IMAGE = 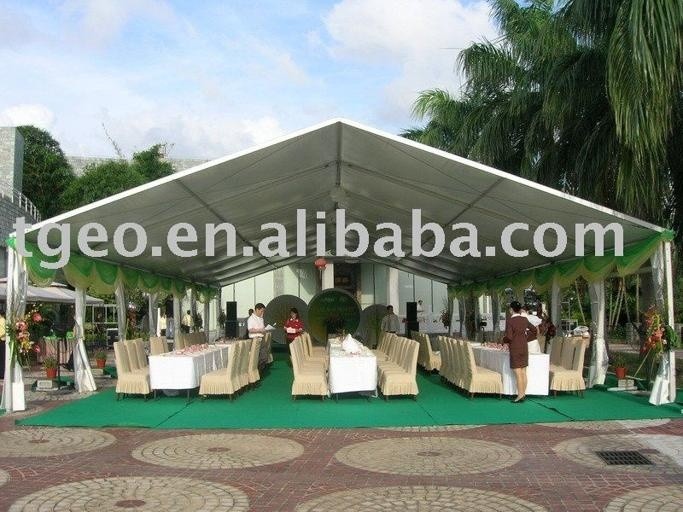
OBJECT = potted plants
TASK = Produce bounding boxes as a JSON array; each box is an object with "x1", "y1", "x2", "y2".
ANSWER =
[
  {"x1": 94, "y1": 351, "x2": 106, "y2": 369},
  {"x1": 42, "y1": 357, "x2": 60, "y2": 378},
  {"x1": 612, "y1": 351, "x2": 628, "y2": 378}
]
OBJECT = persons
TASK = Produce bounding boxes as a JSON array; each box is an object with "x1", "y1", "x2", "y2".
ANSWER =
[
  {"x1": 417, "y1": 300, "x2": 423, "y2": 317},
  {"x1": 501, "y1": 301, "x2": 536, "y2": 403},
  {"x1": 380, "y1": 305, "x2": 400, "y2": 333},
  {"x1": 248, "y1": 309, "x2": 253, "y2": 315},
  {"x1": 284, "y1": 308, "x2": 304, "y2": 366},
  {"x1": 180, "y1": 310, "x2": 194, "y2": 333},
  {"x1": 521, "y1": 306, "x2": 543, "y2": 352},
  {"x1": 247, "y1": 303, "x2": 268, "y2": 373},
  {"x1": 160, "y1": 311, "x2": 167, "y2": 336},
  {"x1": 1, "y1": 310, "x2": 6, "y2": 379}
]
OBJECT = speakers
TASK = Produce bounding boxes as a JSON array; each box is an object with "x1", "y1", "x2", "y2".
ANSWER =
[
  {"x1": 405, "y1": 322, "x2": 419, "y2": 338},
  {"x1": 224, "y1": 321, "x2": 239, "y2": 338},
  {"x1": 406, "y1": 301, "x2": 417, "y2": 321},
  {"x1": 226, "y1": 302, "x2": 237, "y2": 321}
]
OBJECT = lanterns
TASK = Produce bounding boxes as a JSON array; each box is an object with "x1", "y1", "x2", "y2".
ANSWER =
[{"x1": 314, "y1": 257, "x2": 326, "y2": 270}]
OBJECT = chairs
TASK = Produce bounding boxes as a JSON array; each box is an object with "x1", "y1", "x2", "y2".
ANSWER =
[{"x1": 111, "y1": 319, "x2": 594, "y2": 403}]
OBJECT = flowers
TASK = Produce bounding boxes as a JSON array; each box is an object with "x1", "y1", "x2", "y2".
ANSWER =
[
  {"x1": 640, "y1": 305, "x2": 681, "y2": 357},
  {"x1": 7, "y1": 295, "x2": 54, "y2": 365}
]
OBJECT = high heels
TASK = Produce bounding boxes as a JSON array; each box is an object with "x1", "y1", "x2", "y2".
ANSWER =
[{"x1": 509, "y1": 394, "x2": 527, "y2": 403}]
